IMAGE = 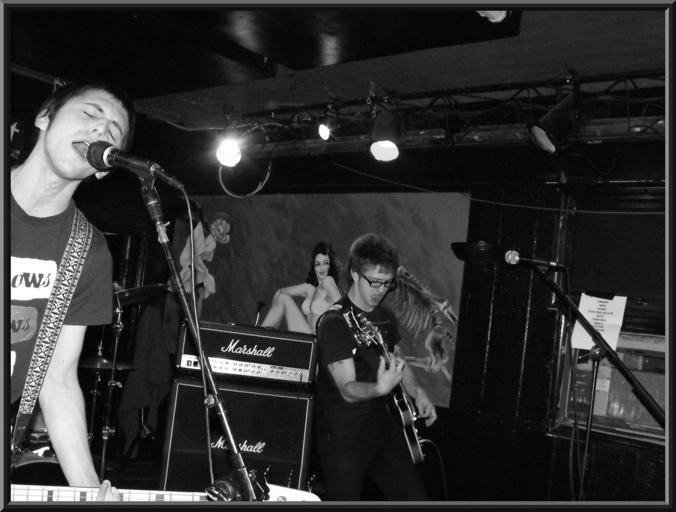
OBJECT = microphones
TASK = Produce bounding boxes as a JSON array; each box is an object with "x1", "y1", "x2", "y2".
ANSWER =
[
  {"x1": 86, "y1": 140, "x2": 161, "y2": 178},
  {"x1": 504, "y1": 250, "x2": 562, "y2": 270}
]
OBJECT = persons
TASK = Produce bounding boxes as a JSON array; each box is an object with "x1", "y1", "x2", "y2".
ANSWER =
[
  {"x1": 178, "y1": 211, "x2": 232, "y2": 322},
  {"x1": 260, "y1": 241, "x2": 343, "y2": 337},
  {"x1": 9, "y1": 76, "x2": 148, "y2": 503},
  {"x1": 310, "y1": 234, "x2": 439, "y2": 500}
]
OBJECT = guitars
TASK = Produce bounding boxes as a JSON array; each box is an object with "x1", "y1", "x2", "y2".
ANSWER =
[
  {"x1": 343, "y1": 306, "x2": 432, "y2": 464},
  {"x1": 10, "y1": 453, "x2": 323, "y2": 503}
]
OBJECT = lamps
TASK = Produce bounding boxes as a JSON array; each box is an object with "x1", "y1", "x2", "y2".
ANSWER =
[{"x1": 175, "y1": 66, "x2": 665, "y2": 202}]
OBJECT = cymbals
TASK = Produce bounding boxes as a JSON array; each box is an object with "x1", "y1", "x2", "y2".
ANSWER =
[
  {"x1": 112, "y1": 283, "x2": 170, "y2": 307},
  {"x1": 80, "y1": 357, "x2": 134, "y2": 371}
]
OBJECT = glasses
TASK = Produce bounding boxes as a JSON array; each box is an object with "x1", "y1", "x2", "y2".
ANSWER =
[{"x1": 358, "y1": 270, "x2": 397, "y2": 289}]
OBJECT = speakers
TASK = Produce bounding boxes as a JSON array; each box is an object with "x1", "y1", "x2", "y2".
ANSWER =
[{"x1": 157, "y1": 377, "x2": 317, "y2": 493}]
